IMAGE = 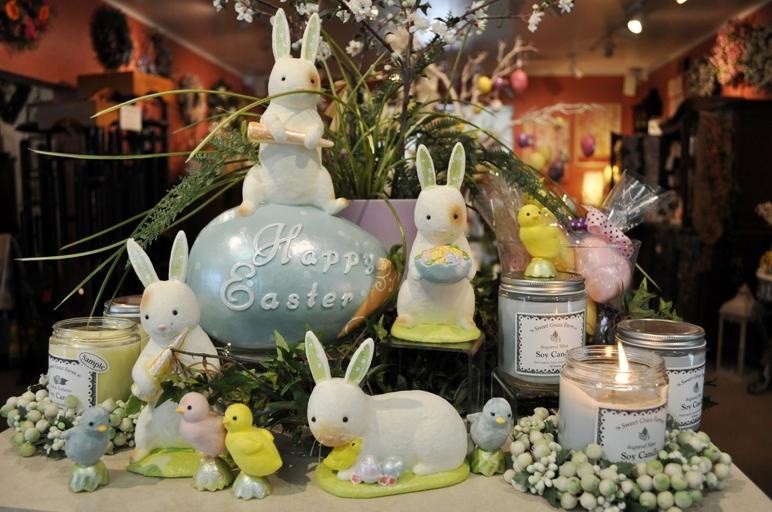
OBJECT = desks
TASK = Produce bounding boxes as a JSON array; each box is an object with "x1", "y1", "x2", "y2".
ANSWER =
[{"x1": 0, "y1": 424, "x2": 771, "y2": 512}]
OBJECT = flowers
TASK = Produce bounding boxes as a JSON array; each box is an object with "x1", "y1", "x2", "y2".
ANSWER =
[
  {"x1": 175, "y1": 73, "x2": 207, "y2": 127},
  {"x1": 0, "y1": 0, "x2": 53, "y2": 52},
  {"x1": 709, "y1": 17, "x2": 770, "y2": 93},
  {"x1": 38, "y1": 1, "x2": 680, "y2": 324}
]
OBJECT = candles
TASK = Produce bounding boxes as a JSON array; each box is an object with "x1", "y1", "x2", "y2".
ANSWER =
[
  {"x1": 49, "y1": 328, "x2": 140, "y2": 415},
  {"x1": 556, "y1": 365, "x2": 666, "y2": 463}
]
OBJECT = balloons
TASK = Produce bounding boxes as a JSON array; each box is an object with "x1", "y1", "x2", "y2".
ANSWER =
[
  {"x1": 477, "y1": 75, "x2": 493, "y2": 93},
  {"x1": 580, "y1": 134, "x2": 596, "y2": 158},
  {"x1": 541, "y1": 144, "x2": 552, "y2": 163},
  {"x1": 529, "y1": 152, "x2": 546, "y2": 171},
  {"x1": 519, "y1": 133, "x2": 534, "y2": 149},
  {"x1": 511, "y1": 70, "x2": 528, "y2": 93},
  {"x1": 548, "y1": 160, "x2": 566, "y2": 181}
]
